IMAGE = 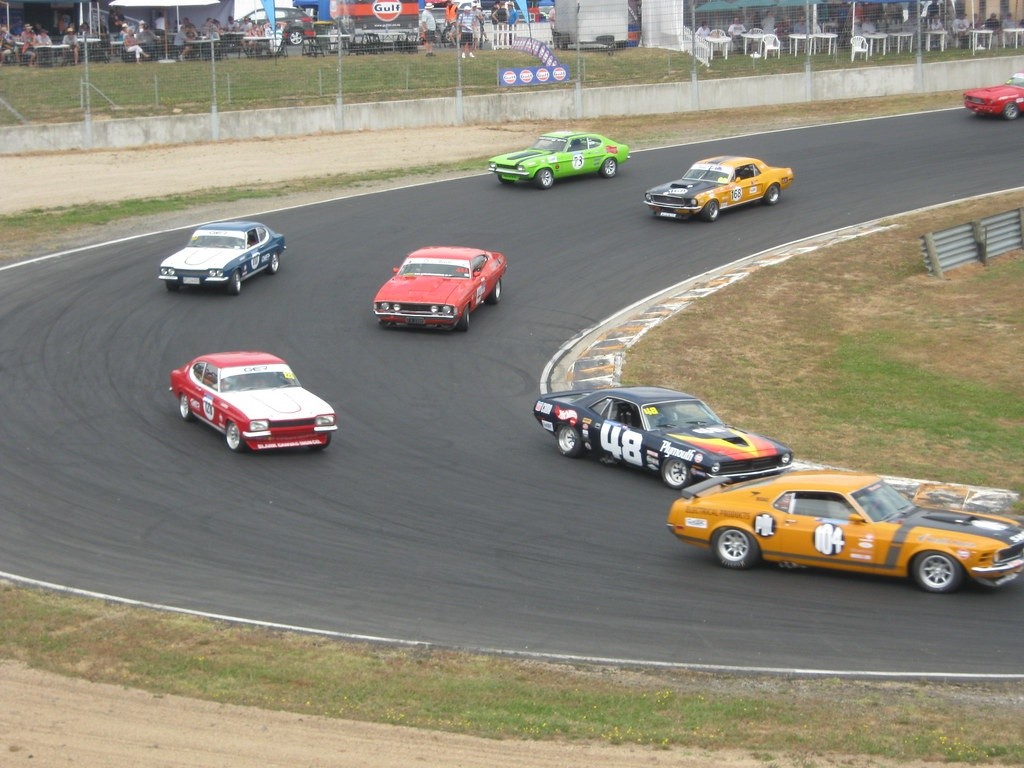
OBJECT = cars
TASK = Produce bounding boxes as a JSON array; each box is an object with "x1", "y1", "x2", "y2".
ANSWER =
[
  {"x1": 159, "y1": 221, "x2": 285, "y2": 295},
  {"x1": 963, "y1": 69, "x2": 1024, "y2": 121},
  {"x1": 418, "y1": 9, "x2": 464, "y2": 43},
  {"x1": 371, "y1": 247, "x2": 507, "y2": 331},
  {"x1": 532, "y1": 385, "x2": 794, "y2": 490},
  {"x1": 487, "y1": 130, "x2": 630, "y2": 189},
  {"x1": 169, "y1": 350, "x2": 337, "y2": 453},
  {"x1": 644, "y1": 155, "x2": 793, "y2": 223},
  {"x1": 666, "y1": 466, "x2": 1024, "y2": 593}
]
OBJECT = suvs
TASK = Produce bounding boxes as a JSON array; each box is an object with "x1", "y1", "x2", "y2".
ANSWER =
[{"x1": 235, "y1": 7, "x2": 317, "y2": 45}]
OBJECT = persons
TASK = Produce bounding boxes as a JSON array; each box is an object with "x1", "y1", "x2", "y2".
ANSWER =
[
  {"x1": 174, "y1": 13, "x2": 253, "y2": 60},
  {"x1": 697, "y1": 21, "x2": 710, "y2": 37},
  {"x1": 155, "y1": 12, "x2": 165, "y2": 34},
  {"x1": 79, "y1": 6, "x2": 154, "y2": 64},
  {"x1": 762, "y1": 11, "x2": 817, "y2": 52},
  {"x1": 728, "y1": 18, "x2": 752, "y2": 53},
  {"x1": 837, "y1": 2, "x2": 902, "y2": 47},
  {"x1": 0, "y1": 20, "x2": 78, "y2": 65},
  {"x1": 445, "y1": 1, "x2": 519, "y2": 58},
  {"x1": 422, "y1": 3, "x2": 436, "y2": 56},
  {"x1": 926, "y1": 0, "x2": 1024, "y2": 50}
]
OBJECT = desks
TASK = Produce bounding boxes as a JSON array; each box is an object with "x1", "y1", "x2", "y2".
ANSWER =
[
  {"x1": 923, "y1": 30, "x2": 949, "y2": 53},
  {"x1": 968, "y1": 29, "x2": 994, "y2": 51},
  {"x1": 220, "y1": 32, "x2": 246, "y2": 59},
  {"x1": 187, "y1": 39, "x2": 221, "y2": 58},
  {"x1": 891, "y1": 32, "x2": 913, "y2": 54},
  {"x1": 1002, "y1": 28, "x2": 1024, "y2": 49},
  {"x1": 375, "y1": 32, "x2": 405, "y2": 54},
  {"x1": 706, "y1": 36, "x2": 732, "y2": 61},
  {"x1": 740, "y1": 33, "x2": 765, "y2": 55},
  {"x1": 243, "y1": 36, "x2": 275, "y2": 59},
  {"x1": 862, "y1": 32, "x2": 888, "y2": 56},
  {"x1": 316, "y1": 34, "x2": 351, "y2": 54},
  {"x1": 813, "y1": 33, "x2": 839, "y2": 56},
  {"x1": 33, "y1": 45, "x2": 71, "y2": 66},
  {"x1": 77, "y1": 37, "x2": 102, "y2": 63},
  {"x1": 788, "y1": 33, "x2": 813, "y2": 57}
]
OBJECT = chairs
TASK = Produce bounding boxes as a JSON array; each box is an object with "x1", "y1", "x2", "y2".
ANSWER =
[
  {"x1": 808, "y1": 494, "x2": 832, "y2": 518},
  {"x1": 618, "y1": 403, "x2": 641, "y2": 428},
  {"x1": 0, "y1": 31, "x2": 421, "y2": 69},
  {"x1": 248, "y1": 235, "x2": 256, "y2": 246},
  {"x1": 708, "y1": 19, "x2": 1014, "y2": 66},
  {"x1": 569, "y1": 139, "x2": 585, "y2": 151}
]
